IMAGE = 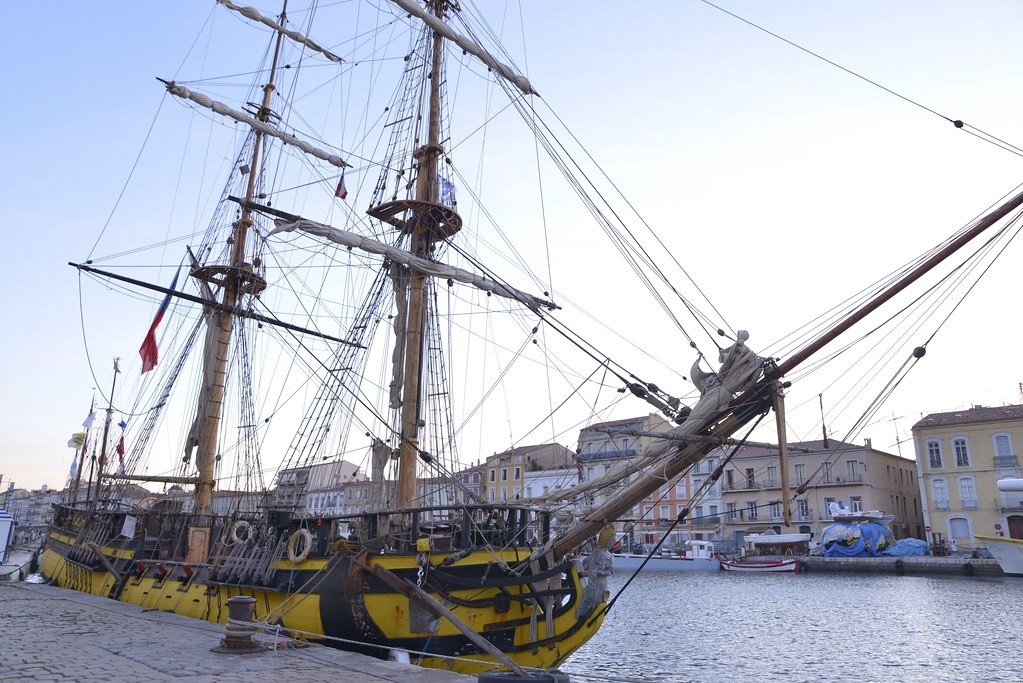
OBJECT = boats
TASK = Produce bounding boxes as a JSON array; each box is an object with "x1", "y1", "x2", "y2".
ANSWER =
[
  {"x1": 973, "y1": 535, "x2": 1023, "y2": 575},
  {"x1": 577, "y1": 540, "x2": 721, "y2": 572},
  {"x1": 718, "y1": 556, "x2": 800, "y2": 572}
]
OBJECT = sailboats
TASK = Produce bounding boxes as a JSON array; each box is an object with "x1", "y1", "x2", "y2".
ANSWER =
[{"x1": 37, "y1": 0, "x2": 1023, "y2": 675}]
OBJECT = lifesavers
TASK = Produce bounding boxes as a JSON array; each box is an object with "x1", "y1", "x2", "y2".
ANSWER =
[
  {"x1": 231, "y1": 521, "x2": 253, "y2": 545},
  {"x1": 287, "y1": 528, "x2": 312, "y2": 562}
]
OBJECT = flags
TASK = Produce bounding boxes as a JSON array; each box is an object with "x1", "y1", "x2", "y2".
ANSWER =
[{"x1": 138, "y1": 260, "x2": 184, "y2": 375}]
